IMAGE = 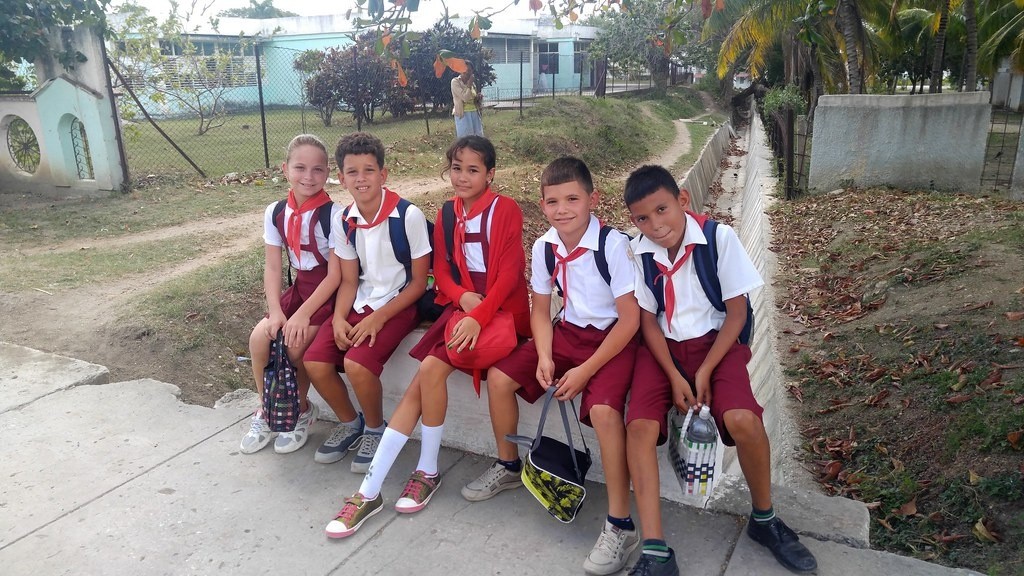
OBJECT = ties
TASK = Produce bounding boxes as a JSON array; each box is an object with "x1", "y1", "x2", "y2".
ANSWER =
[
  {"x1": 654, "y1": 211, "x2": 710, "y2": 334},
  {"x1": 286, "y1": 188, "x2": 332, "y2": 272},
  {"x1": 549, "y1": 218, "x2": 605, "y2": 329},
  {"x1": 339, "y1": 187, "x2": 403, "y2": 247},
  {"x1": 453, "y1": 185, "x2": 497, "y2": 293}
]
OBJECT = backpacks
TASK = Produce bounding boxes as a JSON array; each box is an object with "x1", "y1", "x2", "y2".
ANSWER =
[
  {"x1": 638, "y1": 219, "x2": 756, "y2": 348},
  {"x1": 341, "y1": 198, "x2": 446, "y2": 322}
]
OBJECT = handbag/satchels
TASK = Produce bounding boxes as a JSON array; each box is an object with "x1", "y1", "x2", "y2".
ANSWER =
[
  {"x1": 262, "y1": 327, "x2": 300, "y2": 432},
  {"x1": 667, "y1": 401, "x2": 719, "y2": 494},
  {"x1": 503, "y1": 375, "x2": 592, "y2": 523},
  {"x1": 445, "y1": 295, "x2": 517, "y2": 369}
]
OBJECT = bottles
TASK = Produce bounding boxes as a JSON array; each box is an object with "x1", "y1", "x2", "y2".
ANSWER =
[{"x1": 688, "y1": 405, "x2": 716, "y2": 444}]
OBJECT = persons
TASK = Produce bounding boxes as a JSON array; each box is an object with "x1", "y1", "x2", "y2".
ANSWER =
[
  {"x1": 460, "y1": 156, "x2": 641, "y2": 576},
  {"x1": 236, "y1": 134, "x2": 342, "y2": 455},
  {"x1": 325, "y1": 135, "x2": 531, "y2": 539},
  {"x1": 623, "y1": 164, "x2": 818, "y2": 576},
  {"x1": 451, "y1": 59, "x2": 485, "y2": 138},
  {"x1": 300, "y1": 131, "x2": 431, "y2": 475}
]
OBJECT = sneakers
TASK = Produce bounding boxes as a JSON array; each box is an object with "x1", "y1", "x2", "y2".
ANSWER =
[
  {"x1": 324, "y1": 491, "x2": 384, "y2": 539},
  {"x1": 747, "y1": 516, "x2": 819, "y2": 573},
  {"x1": 583, "y1": 517, "x2": 640, "y2": 576},
  {"x1": 459, "y1": 460, "x2": 524, "y2": 502},
  {"x1": 314, "y1": 411, "x2": 365, "y2": 463},
  {"x1": 350, "y1": 420, "x2": 389, "y2": 474},
  {"x1": 274, "y1": 396, "x2": 319, "y2": 453},
  {"x1": 394, "y1": 469, "x2": 442, "y2": 513},
  {"x1": 628, "y1": 547, "x2": 680, "y2": 576},
  {"x1": 240, "y1": 408, "x2": 277, "y2": 454}
]
